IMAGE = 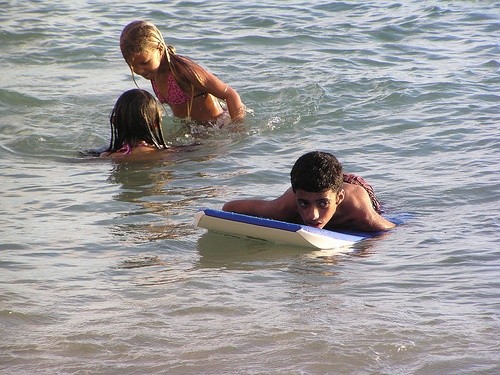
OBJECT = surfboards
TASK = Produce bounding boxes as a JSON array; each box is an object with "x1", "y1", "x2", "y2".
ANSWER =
[{"x1": 194, "y1": 209, "x2": 373, "y2": 249}]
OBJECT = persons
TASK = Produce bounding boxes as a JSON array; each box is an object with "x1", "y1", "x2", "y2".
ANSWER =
[
  {"x1": 77, "y1": 88, "x2": 176, "y2": 161},
  {"x1": 222, "y1": 151, "x2": 416, "y2": 235},
  {"x1": 120, "y1": 19, "x2": 245, "y2": 130}
]
